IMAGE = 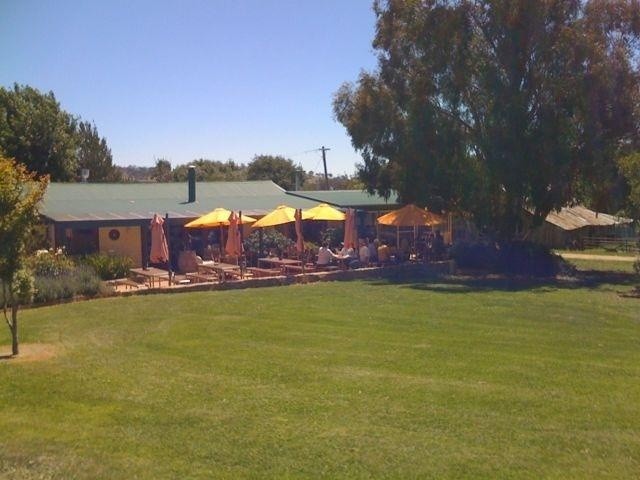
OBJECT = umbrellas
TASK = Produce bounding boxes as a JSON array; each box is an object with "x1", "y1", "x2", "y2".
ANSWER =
[
  {"x1": 305, "y1": 202, "x2": 345, "y2": 244},
  {"x1": 376, "y1": 203, "x2": 448, "y2": 246},
  {"x1": 344, "y1": 207, "x2": 360, "y2": 250},
  {"x1": 147, "y1": 214, "x2": 171, "y2": 264},
  {"x1": 184, "y1": 208, "x2": 257, "y2": 257},
  {"x1": 226, "y1": 211, "x2": 242, "y2": 259},
  {"x1": 294, "y1": 209, "x2": 305, "y2": 256},
  {"x1": 250, "y1": 205, "x2": 313, "y2": 258}
]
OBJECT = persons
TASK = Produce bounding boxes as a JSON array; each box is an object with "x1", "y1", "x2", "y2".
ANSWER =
[{"x1": 315, "y1": 230, "x2": 446, "y2": 271}]
